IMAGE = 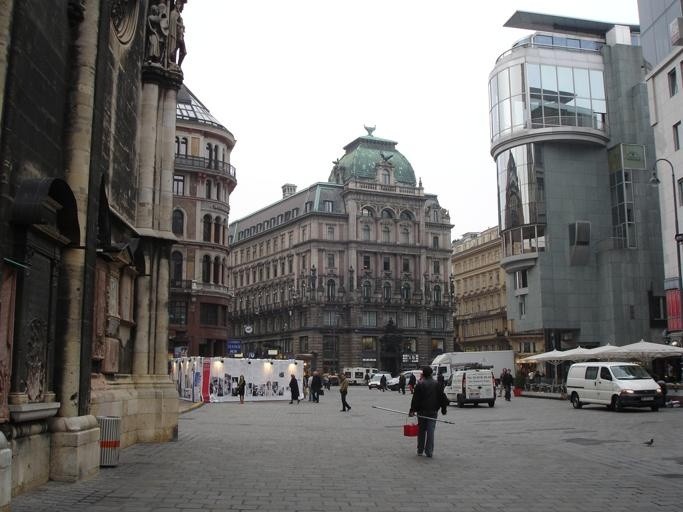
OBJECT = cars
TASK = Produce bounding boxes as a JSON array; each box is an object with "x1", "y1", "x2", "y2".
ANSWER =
[{"x1": 340, "y1": 366, "x2": 422, "y2": 390}]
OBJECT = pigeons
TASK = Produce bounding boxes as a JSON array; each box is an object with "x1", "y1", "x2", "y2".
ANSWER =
[{"x1": 643, "y1": 438, "x2": 654, "y2": 447}]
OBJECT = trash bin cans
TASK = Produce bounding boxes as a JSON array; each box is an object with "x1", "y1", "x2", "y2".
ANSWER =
[{"x1": 96, "y1": 415, "x2": 121, "y2": 468}]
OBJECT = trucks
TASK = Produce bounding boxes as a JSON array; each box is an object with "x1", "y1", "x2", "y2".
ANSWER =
[{"x1": 431, "y1": 351, "x2": 516, "y2": 382}]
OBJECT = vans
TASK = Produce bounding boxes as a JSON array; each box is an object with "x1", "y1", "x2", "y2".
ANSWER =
[
  {"x1": 566, "y1": 362, "x2": 664, "y2": 412},
  {"x1": 444, "y1": 370, "x2": 496, "y2": 408}
]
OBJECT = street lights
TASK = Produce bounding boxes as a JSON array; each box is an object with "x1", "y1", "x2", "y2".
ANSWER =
[{"x1": 650, "y1": 158, "x2": 682, "y2": 310}]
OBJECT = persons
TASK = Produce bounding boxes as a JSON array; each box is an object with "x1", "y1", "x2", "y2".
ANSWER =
[
  {"x1": 212, "y1": 378, "x2": 219, "y2": 397},
  {"x1": 534, "y1": 370, "x2": 545, "y2": 384},
  {"x1": 233, "y1": 378, "x2": 238, "y2": 393},
  {"x1": 497, "y1": 368, "x2": 507, "y2": 397},
  {"x1": 503, "y1": 368, "x2": 514, "y2": 401},
  {"x1": 666, "y1": 362, "x2": 674, "y2": 382},
  {"x1": 408, "y1": 366, "x2": 448, "y2": 458},
  {"x1": 365, "y1": 371, "x2": 370, "y2": 385},
  {"x1": 264, "y1": 381, "x2": 277, "y2": 397},
  {"x1": 288, "y1": 370, "x2": 332, "y2": 404},
  {"x1": 398, "y1": 371, "x2": 445, "y2": 394},
  {"x1": 339, "y1": 372, "x2": 351, "y2": 412},
  {"x1": 237, "y1": 374, "x2": 246, "y2": 404},
  {"x1": 380, "y1": 374, "x2": 387, "y2": 392},
  {"x1": 528, "y1": 369, "x2": 535, "y2": 382}
]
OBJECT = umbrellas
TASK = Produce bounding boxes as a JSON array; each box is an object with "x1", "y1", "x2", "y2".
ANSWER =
[
  {"x1": 591, "y1": 341, "x2": 619, "y2": 362},
  {"x1": 607, "y1": 337, "x2": 683, "y2": 366},
  {"x1": 536, "y1": 344, "x2": 596, "y2": 365},
  {"x1": 516, "y1": 348, "x2": 568, "y2": 385}
]
